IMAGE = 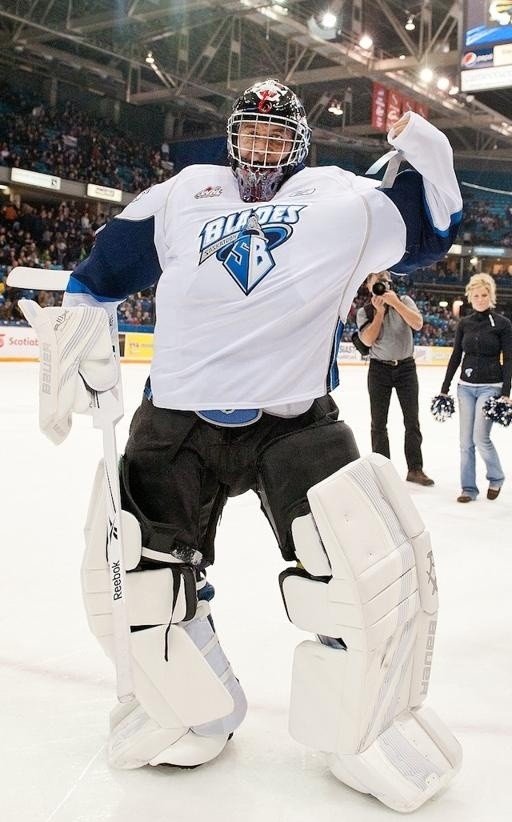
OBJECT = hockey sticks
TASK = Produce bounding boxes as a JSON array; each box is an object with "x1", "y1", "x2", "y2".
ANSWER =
[{"x1": 8, "y1": 268, "x2": 136, "y2": 717}]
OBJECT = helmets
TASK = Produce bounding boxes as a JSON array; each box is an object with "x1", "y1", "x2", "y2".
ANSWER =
[{"x1": 227, "y1": 80, "x2": 312, "y2": 166}]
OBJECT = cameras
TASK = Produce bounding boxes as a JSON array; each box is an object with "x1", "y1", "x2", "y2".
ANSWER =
[{"x1": 372, "y1": 281, "x2": 389, "y2": 296}]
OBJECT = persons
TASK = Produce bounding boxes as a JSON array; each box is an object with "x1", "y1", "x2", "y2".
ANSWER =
[
  {"x1": 438, "y1": 272, "x2": 512, "y2": 503},
  {"x1": 355, "y1": 268, "x2": 435, "y2": 486},
  {"x1": 18, "y1": 79, "x2": 465, "y2": 812},
  {"x1": 1, "y1": 90, "x2": 512, "y2": 346}
]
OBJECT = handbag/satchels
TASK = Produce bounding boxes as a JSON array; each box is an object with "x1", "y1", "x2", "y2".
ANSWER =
[{"x1": 352, "y1": 320, "x2": 369, "y2": 355}]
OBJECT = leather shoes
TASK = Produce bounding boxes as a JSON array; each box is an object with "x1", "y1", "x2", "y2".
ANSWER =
[{"x1": 406, "y1": 471, "x2": 434, "y2": 486}]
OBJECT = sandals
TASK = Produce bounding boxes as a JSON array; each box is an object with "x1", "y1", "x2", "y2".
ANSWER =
[
  {"x1": 488, "y1": 485, "x2": 500, "y2": 500},
  {"x1": 457, "y1": 496, "x2": 471, "y2": 503}
]
